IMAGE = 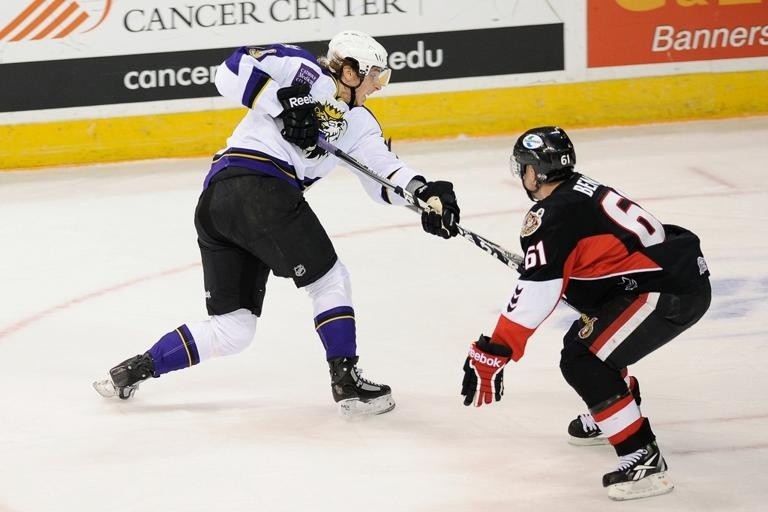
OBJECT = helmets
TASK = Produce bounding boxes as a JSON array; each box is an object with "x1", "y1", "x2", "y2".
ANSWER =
[
  {"x1": 510, "y1": 127, "x2": 576, "y2": 184},
  {"x1": 327, "y1": 31, "x2": 392, "y2": 87}
]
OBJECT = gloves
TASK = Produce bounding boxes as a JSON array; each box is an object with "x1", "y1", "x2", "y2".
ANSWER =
[
  {"x1": 415, "y1": 181, "x2": 459, "y2": 239},
  {"x1": 277, "y1": 85, "x2": 319, "y2": 150},
  {"x1": 461, "y1": 335, "x2": 512, "y2": 407}
]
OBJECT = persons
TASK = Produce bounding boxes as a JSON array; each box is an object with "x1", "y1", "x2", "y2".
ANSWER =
[
  {"x1": 110, "y1": 29, "x2": 456, "y2": 407},
  {"x1": 460, "y1": 126, "x2": 713, "y2": 489}
]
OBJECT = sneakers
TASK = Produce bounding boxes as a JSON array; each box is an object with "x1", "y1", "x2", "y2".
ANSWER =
[
  {"x1": 568, "y1": 406, "x2": 642, "y2": 438},
  {"x1": 603, "y1": 440, "x2": 667, "y2": 487}
]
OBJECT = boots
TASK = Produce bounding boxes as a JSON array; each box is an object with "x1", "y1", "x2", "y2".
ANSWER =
[
  {"x1": 110, "y1": 351, "x2": 152, "y2": 399},
  {"x1": 328, "y1": 356, "x2": 390, "y2": 402}
]
